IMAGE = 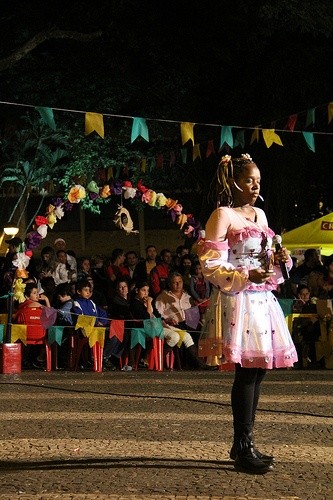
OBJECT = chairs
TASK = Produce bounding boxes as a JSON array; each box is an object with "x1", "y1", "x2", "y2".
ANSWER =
[{"x1": 11, "y1": 307, "x2": 175, "y2": 372}]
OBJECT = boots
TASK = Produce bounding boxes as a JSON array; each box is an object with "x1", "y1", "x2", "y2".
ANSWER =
[
  {"x1": 230, "y1": 421, "x2": 276, "y2": 463},
  {"x1": 234, "y1": 430, "x2": 268, "y2": 475}
]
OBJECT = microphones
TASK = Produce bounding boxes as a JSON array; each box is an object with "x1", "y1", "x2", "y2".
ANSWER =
[{"x1": 273, "y1": 235, "x2": 290, "y2": 280}]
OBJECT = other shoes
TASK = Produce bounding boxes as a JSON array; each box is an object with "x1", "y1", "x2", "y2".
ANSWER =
[
  {"x1": 121, "y1": 365, "x2": 126, "y2": 371},
  {"x1": 126, "y1": 366, "x2": 133, "y2": 371},
  {"x1": 204, "y1": 365, "x2": 218, "y2": 370},
  {"x1": 29, "y1": 363, "x2": 39, "y2": 369},
  {"x1": 112, "y1": 367, "x2": 118, "y2": 370}
]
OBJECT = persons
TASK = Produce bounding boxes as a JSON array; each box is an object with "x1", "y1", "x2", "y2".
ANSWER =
[
  {"x1": 198, "y1": 154, "x2": 299, "y2": 473},
  {"x1": 275, "y1": 248, "x2": 333, "y2": 370},
  {"x1": 0, "y1": 237, "x2": 210, "y2": 372}
]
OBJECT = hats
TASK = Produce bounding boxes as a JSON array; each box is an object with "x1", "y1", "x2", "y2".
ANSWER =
[{"x1": 54, "y1": 238, "x2": 65, "y2": 245}]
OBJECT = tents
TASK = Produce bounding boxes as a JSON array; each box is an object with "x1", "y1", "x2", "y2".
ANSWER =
[{"x1": 271, "y1": 211, "x2": 333, "y2": 256}]
